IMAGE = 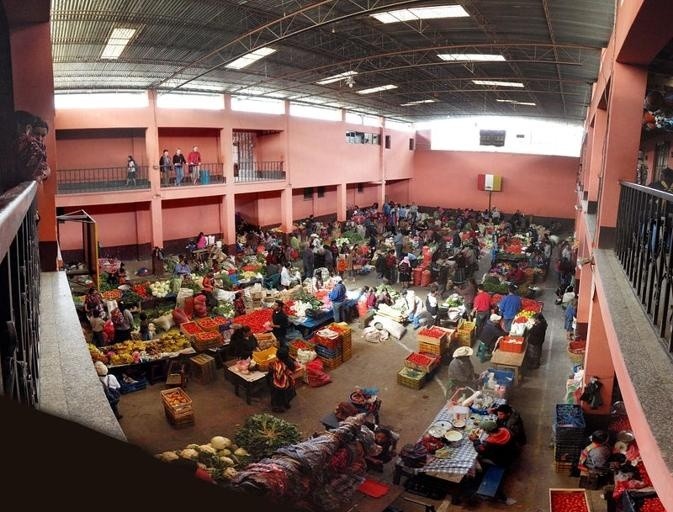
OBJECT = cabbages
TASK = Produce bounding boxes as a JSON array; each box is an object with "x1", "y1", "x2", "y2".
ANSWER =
[
  {"x1": 159, "y1": 436, "x2": 248, "y2": 480},
  {"x1": 149, "y1": 280, "x2": 172, "y2": 298}
]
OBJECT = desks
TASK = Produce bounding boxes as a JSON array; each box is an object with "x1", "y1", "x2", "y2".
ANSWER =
[
  {"x1": 225, "y1": 357, "x2": 271, "y2": 406},
  {"x1": 490, "y1": 342, "x2": 528, "y2": 385},
  {"x1": 319, "y1": 401, "x2": 366, "y2": 431},
  {"x1": 192, "y1": 249, "x2": 210, "y2": 261},
  {"x1": 393, "y1": 393, "x2": 508, "y2": 497},
  {"x1": 331, "y1": 477, "x2": 405, "y2": 512}
]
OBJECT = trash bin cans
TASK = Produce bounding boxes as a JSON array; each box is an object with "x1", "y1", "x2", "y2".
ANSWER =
[
  {"x1": 200, "y1": 170, "x2": 209, "y2": 184},
  {"x1": 152, "y1": 246, "x2": 164, "y2": 274}
]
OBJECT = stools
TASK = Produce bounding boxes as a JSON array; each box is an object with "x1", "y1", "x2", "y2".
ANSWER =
[{"x1": 475, "y1": 341, "x2": 490, "y2": 363}]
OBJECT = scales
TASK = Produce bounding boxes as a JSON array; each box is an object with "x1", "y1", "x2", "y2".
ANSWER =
[{"x1": 613, "y1": 429, "x2": 636, "y2": 455}]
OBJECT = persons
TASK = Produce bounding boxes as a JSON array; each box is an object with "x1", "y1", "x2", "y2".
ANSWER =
[
  {"x1": 602, "y1": 453, "x2": 642, "y2": 512},
  {"x1": 637, "y1": 150, "x2": 673, "y2": 258},
  {"x1": 172, "y1": 147, "x2": 186, "y2": 186},
  {"x1": 1, "y1": 111, "x2": 51, "y2": 195},
  {"x1": 32, "y1": 114, "x2": 49, "y2": 143},
  {"x1": 186, "y1": 145, "x2": 202, "y2": 184},
  {"x1": 125, "y1": 155, "x2": 138, "y2": 186},
  {"x1": 577, "y1": 430, "x2": 615, "y2": 488},
  {"x1": 160, "y1": 149, "x2": 173, "y2": 184},
  {"x1": 83, "y1": 199, "x2": 572, "y2": 469}
]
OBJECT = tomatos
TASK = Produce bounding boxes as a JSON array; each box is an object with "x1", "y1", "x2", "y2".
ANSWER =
[
  {"x1": 640, "y1": 497, "x2": 666, "y2": 512},
  {"x1": 135, "y1": 286, "x2": 145, "y2": 296},
  {"x1": 407, "y1": 354, "x2": 431, "y2": 365},
  {"x1": 552, "y1": 493, "x2": 588, "y2": 512},
  {"x1": 608, "y1": 415, "x2": 652, "y2": 490},
  {"x1": 489, "y1": 296, "x2": 541, "y2": 313},
  {"x1": 329, "y1": 325, "x2": 345, "y2": 333},
  {"x1": 183, "y1": 316, "x2": 226, "y2": 341},
  {"x1": 234, "y1": 308, "x2": 273, "y2": 334},
  {"x1": 420, "y1": 328, "x2": 442, "y2": 338},
  {"x1": 292, "y1": 340, "x2": 308, "y2": 348}
]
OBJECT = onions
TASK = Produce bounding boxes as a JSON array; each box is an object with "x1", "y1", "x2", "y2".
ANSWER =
[{"x1": 424, "y1": 433, "x2": 443, "y2": 451}]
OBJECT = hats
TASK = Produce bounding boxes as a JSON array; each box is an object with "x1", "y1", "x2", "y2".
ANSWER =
[
  {"x1": 95, "y1": 361, "x2": 108, "y2": 376},
  {"x1": 489, "y1": 314, "x2": 501, "y2": 321},
  {"x1": 480, "y1": 422, "x2": 497, "y2": 432}
]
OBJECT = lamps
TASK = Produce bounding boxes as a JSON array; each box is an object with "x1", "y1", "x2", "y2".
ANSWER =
[
  {"x1": 575, "y1": 253, "x2": 595, "y2": 269},
  {"x1": 575, "y1": 204, "x2": 582, "y2": 212}
]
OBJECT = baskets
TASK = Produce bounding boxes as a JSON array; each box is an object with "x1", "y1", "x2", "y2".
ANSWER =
[
  {"x1": 396, "y1": 322, "x2": 475, "y2": 390},
  {"x1": 119, "y1": 376, "x2": 145, "y2": 394},
  {"x1": 254, "y1": 322, "x2": 351, "y2": 371},
  {"x1": 552, "y1": 404, "x2": 585, "y2": 473},
  {"x1": 160, "y1": 388, "x2": 194, "y2": 430},
  {"x1": 181, "y1": 317, "x2": 231, "y2": 352}
]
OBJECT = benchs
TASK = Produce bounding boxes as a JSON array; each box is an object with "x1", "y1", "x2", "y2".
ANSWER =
[{"x1": 476, "y1": 467, "x2": 505, "y2": 498}]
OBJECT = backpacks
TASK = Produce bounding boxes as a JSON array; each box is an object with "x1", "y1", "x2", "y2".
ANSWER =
[{"x1": 99, "y1": 376, "x2": 119, "y2": 406}]
